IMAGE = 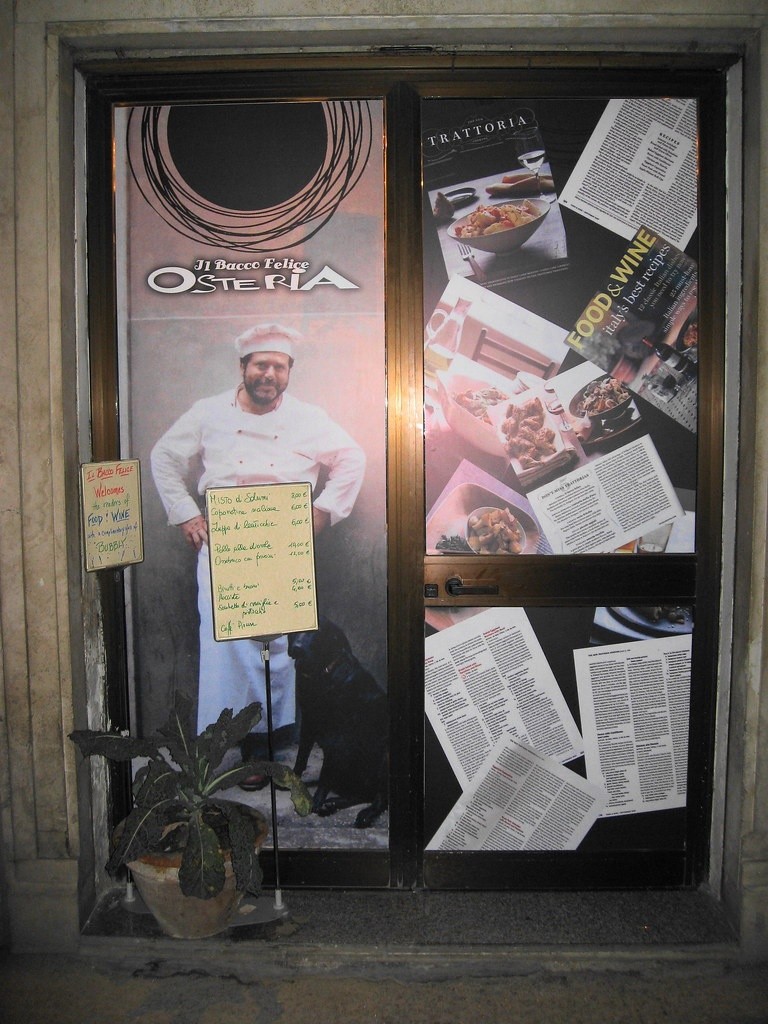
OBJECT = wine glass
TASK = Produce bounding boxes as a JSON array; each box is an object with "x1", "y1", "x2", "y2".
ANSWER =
[
  {"x1": 512, "y1": 127, "x2": 556, "y2": 203},
  {"x1": 544, "y1": 393, "x2": 573, "y2": 431},
  {"x1": 652, "y1": 367, "x2": 691, "y2": 398}
]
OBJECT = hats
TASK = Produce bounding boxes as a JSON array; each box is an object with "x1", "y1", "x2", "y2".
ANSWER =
[{"x1": 236, "y1": 322, "x2": 303, "y2": 357}]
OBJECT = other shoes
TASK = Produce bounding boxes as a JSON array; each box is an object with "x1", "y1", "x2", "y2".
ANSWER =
[{"x1": 239, "y1": 758, "x2": 267, "y2": 791}]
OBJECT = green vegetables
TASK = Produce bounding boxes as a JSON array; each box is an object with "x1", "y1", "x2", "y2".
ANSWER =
[{"x1": 435, "y1": 534, "x2": 473, "y2": 551}]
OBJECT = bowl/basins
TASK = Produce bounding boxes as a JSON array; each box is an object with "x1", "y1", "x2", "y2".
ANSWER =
[
  {"x1": 447, "y1": 198, "x2": 551, "y2": 256},
  {"x1": 436, "y1": 372, "x2": 510, "y2": 457},
  {"x1": 514, "y1": 372, "x2": 545, "y2": 392},
  {"x1": 676, "y1": 306, "x2": 697, "y2": 353},
  {"x1": 465, "y1": 507, "x2": 526, "y2": 555},
  {"x1": 569, "y1": 373, "x2": 633, "y2": 420}
]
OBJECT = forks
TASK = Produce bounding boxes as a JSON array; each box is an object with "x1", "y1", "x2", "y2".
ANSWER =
[{"x1": 458, "y1": 243, "x2": 488, "y2": 282}]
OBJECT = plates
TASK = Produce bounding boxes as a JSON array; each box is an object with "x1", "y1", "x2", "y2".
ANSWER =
[
  {"x1": 444, "y1": 187, "x2": 477, "y2": 208},
  {"x1": 610, "y1": 607, "x2": 693, "y2": 633}
]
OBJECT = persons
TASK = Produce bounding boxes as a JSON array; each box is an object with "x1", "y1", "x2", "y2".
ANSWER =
[{"x1": 149, "y1": 325, "x2": 367, "y2": 792}]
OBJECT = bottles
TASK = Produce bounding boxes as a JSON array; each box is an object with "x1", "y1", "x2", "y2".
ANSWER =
[
  {"x1": 642, "y1": 336, "x2": 697, "y2": 378},
  {"x1": 424, "y1": 297, "x2": 473, "y2": 379}
]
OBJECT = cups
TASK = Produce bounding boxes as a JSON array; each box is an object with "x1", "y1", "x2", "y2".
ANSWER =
[
  {"x1": 637, "y1": 522, "x2": 673, "y2": 553},
  {"x1": 614, "y1": 540, "x2": 635, "y2": 553}
]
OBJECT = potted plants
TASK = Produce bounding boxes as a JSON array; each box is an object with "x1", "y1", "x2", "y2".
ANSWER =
[{"x1": 106, "y1": 701, "x2": 316, "y2": 938}]
